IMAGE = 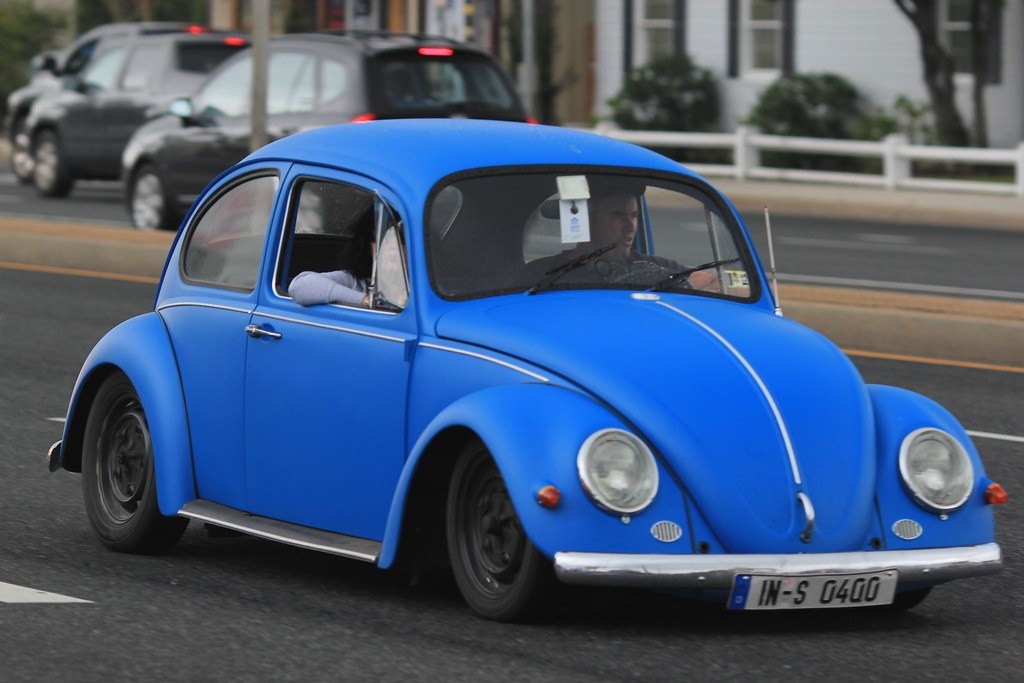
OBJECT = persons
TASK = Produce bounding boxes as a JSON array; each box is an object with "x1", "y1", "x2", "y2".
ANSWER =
[
  {"x1": 283, "y1": 202, "x2": 439, "y2": 309},
  {"x1": 518, "y1": 192, "x2": 716, "y2": 292}
]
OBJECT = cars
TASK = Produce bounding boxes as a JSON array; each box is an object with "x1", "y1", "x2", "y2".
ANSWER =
[
  {"x1": 3, "y1": 20, "x2": 210, "y2": 189},
  {"x1": 121, "y1": 31, "x2": 541, "y2": 233},
  {"x1": 23, "y1": 33, "x2": 257, "y2": 197}
]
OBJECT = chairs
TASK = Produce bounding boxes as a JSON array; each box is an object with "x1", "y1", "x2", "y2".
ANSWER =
[{"x1": 278, "y1": 233, "x2": 351, "y2": 291}]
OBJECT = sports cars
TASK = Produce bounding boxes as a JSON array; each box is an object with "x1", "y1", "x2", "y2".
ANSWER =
[{"x1": 47, "y1": 119, "x2": 1011, "y2": 626}]
